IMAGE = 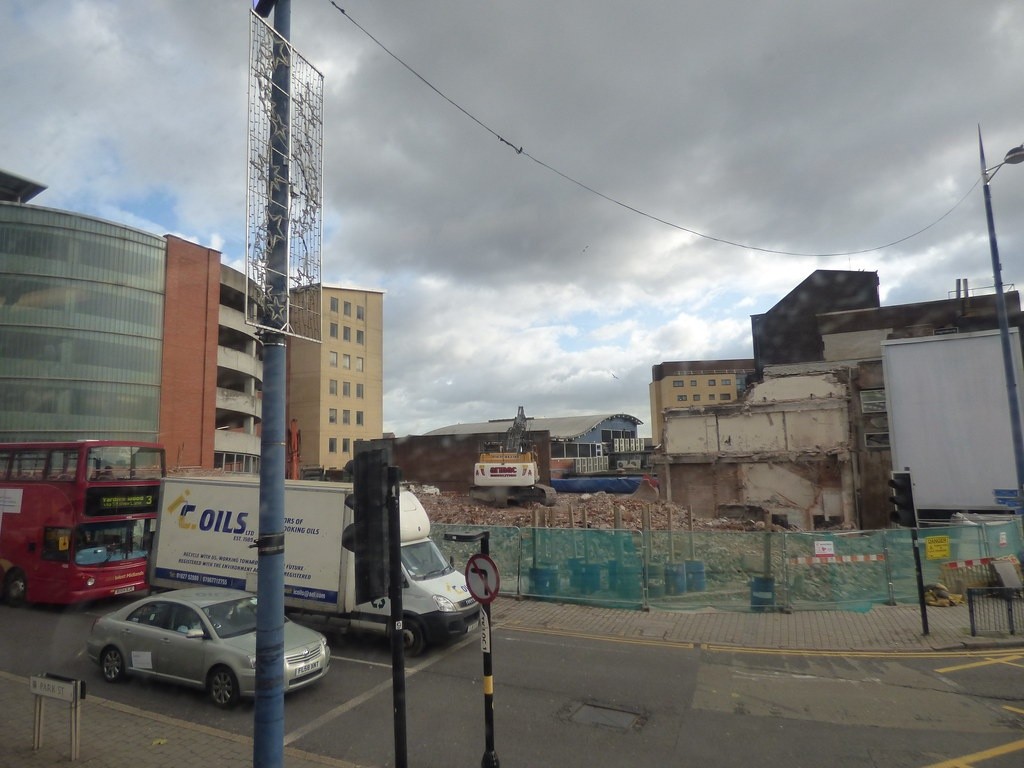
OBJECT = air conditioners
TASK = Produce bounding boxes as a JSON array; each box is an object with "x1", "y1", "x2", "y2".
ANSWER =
[
  {"x1": 612, "y1": 438, "x2": 645, "y2": 453},
  {"x1": 617, "y1": 460, "x2": 627, "y2": 470},
  {"x1": 573, "y1": 455, "x2": 609, "y2": 473},
  {"x1": 630, "y1": 460, "x2": 641, "y2": 469}
]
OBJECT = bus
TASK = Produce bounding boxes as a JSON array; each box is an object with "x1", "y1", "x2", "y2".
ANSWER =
[{"x1": 1, "y1": 438, "x2": 166, "y2": 611}]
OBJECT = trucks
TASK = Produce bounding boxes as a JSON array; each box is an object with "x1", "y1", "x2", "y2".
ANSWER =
[{"x1": 147, "y1": 473, "x2": 481, "y2": 660}]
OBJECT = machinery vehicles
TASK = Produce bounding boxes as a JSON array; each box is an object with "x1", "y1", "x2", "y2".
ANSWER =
[{"x1": 469, "y1": 406, "x2": 558, "y2": 509}]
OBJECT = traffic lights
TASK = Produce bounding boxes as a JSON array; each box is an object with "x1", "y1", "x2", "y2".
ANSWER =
[
  {"x1": 341, "y1": 436, "x2": 397, "y2": 605},
  {"x1": 887, "y1": 470, "x2": 920, "y2": 530}
]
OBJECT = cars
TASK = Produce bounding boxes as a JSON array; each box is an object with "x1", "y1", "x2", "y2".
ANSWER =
[{"x1": 88, "y1": 586, "x2": 331, "y2": 709}]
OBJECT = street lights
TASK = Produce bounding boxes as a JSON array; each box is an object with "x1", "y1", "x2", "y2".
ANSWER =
[
  {"x1": 976, "y1": 122, "x2": 1023, "y2": 507},
  {"x1": 443, "y1": 529, "x2": 502, "y2": 768}
]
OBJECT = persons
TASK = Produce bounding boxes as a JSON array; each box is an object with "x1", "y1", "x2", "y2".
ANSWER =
[{"x1": 94, "y1": 466, "x2": 115, "y2": 481}]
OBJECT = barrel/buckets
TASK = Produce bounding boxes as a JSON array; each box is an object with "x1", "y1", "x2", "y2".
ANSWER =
[
  {"x1": 750, "y1": 575, "x2": 775, "y2": 611},
  {"x1": 529, "y1": 556, "x2": 706, "y2": 598}
]
